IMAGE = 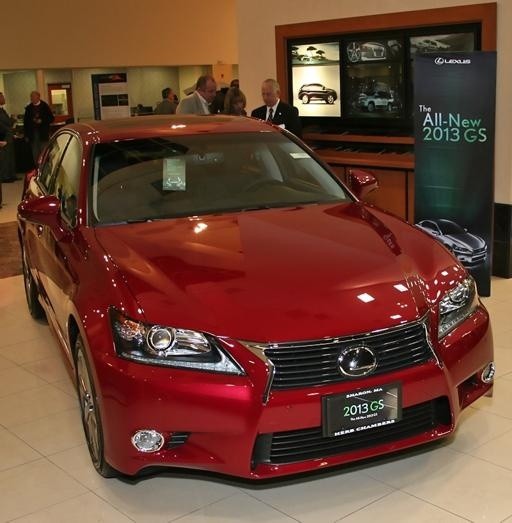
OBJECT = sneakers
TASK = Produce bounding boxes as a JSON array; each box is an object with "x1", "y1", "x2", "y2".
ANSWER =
[{"x1": 2, "y1": 175, "x2": 22, "y2": 183}]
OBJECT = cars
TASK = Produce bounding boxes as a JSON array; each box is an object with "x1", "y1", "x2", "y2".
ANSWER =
[
  {"x1": 17, "y1": 116, "x2": 496, "y2": 483},
  {"x1": 413, "y1": 218, "x2": 487, "y2": 264}
]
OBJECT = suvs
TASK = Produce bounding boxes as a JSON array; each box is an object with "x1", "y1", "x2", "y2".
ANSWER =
[{"x1": 292, "y1": 40, "x2": 450, "y2": 111}]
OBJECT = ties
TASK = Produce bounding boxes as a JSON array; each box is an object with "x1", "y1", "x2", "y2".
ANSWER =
[{"x1": 267, "y1": 107, "x2": 274, "y2": 123}]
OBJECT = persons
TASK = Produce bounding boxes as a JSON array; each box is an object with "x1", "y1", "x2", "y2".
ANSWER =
[
  {"x1": 152, "y1": 74, "x2": 299, "y2": 139},
  {"x1": 22, "y1": 91, "x2": 56, "y2": 169},
  {"x1": 1, "y1": 92, "x2": 23, "y2": 184}
]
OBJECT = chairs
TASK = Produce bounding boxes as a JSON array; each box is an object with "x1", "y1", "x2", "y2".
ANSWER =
[{"x1": 100, "y1": 154, "x2": 279, "y2": 202}]
